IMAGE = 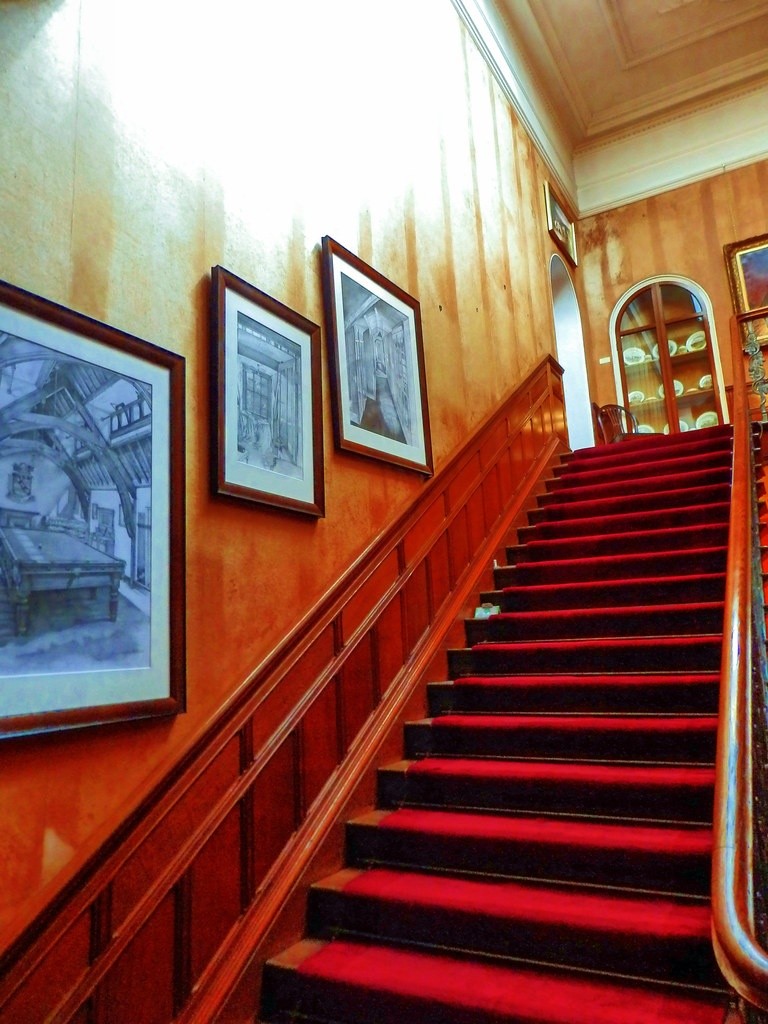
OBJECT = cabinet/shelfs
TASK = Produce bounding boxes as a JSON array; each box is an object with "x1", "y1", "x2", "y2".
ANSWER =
[{"x1": 624, "y1": 347, "x2": 717, "y2": 434}]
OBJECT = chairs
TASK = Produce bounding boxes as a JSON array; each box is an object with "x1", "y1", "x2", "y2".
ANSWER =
[{"x1": 597, "y1": 404, "x2": 638, "y2": 444}]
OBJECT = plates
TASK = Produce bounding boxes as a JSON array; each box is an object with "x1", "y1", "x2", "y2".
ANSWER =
[
  {"x1": 628, "y1": 390, "x2": 644, "y2": 402},
  {"x1": 652, "y1": 339, "x2": 677, "y2": 359},
  {"x1": 659, "y1": 379, "x2": 683, "y2": 398},
  {"x1": 696, "y1": 412, "x2": 719, "y2": 429},
  {"x1": 700, "y1": 374, "x2": 712, "y2": 388},
  {"x1": 632, "y1": 424, "x2": 653, "y2": 433},
  {"x1": 646, "y1": 396, "x2": 658, "y2": 402},
  {"x1": 684, "y1": 388, "x2": 699, "y2": 395},
  {"x1": 686, "y1": 331, "x2": 707, "y2": 352},
  {"x1": 622, "y1": 348, "x2": 646, "y2": 365},
  {"x1": 664, "y1": 420, "x2": 688, "y2": 436}
]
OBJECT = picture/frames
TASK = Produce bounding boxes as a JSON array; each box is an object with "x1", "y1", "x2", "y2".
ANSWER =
[
  {"x1": 545, "y1": 181, "x2": 578, "y2": 267},
  {"x1": 723, "y1": 233, "x2": 768, "y2": 355},
  {"x1": 0, "y1": 278, "x2": 186, "y2": 740},
  {"x1": 208, "y1": 264, "x2": 326, "y2": 521},
  {"x1": 321, "y1": 235, "x2": 434, "y2": 480}
]
